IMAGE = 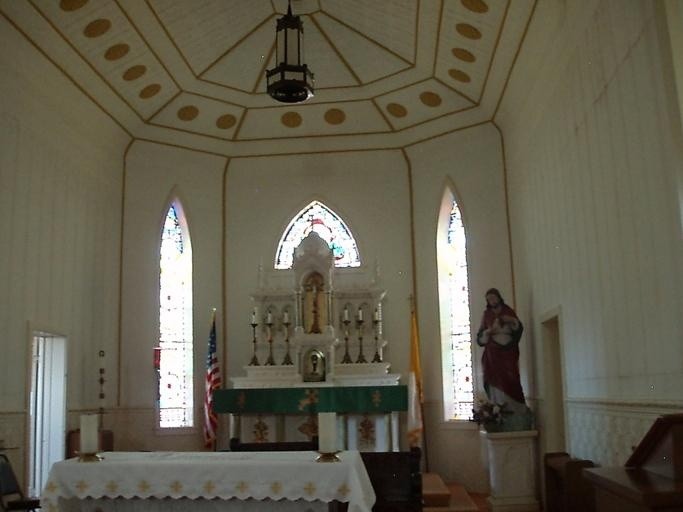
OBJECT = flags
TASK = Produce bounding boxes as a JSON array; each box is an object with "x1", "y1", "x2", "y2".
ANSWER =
[
  {"x1": 203, "y1": 308, "x2": 222, "y2": 452},
  {"x1": 402, "y1": 295, "x2": 427, "y2": 472}
]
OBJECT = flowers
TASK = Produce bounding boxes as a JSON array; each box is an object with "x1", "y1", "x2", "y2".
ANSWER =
[{"x1": 467, "y1": 390, "x2": 514, "y2": 426}]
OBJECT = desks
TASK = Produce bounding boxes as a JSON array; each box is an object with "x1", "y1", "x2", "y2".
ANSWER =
[{"x1": 39, "y1": 451, "x2": 376, "y2": 512}]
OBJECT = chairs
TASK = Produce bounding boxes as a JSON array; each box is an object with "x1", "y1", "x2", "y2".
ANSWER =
[
  {"x1": 332, "y1": 446, "x2": 424, "y2": 512},
  {"x1": 0, "y1": 453, "x2": 40, "y2": 512},
  {"x1": 229, "y1": 435, "x2": 320, "y2": 452}
]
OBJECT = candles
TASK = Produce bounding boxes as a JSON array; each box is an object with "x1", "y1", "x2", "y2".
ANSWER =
[
  {"x1": 250, "y1": 306, "x2": 380, "y2": 324},
  {"x1": 317, "y1": 411, "x2": 338, "y2": 452},
  {"x1": 80, "y1": 413, "x2": 99, "y2": 453}
]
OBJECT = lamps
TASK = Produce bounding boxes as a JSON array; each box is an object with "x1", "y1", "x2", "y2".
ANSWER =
[{"x1": 265, "y1": 0, "x2": 316, "y2": 107}]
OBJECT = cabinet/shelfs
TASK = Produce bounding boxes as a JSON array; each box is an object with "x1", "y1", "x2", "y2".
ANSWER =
[
  {"x1": 543, "y1": 452, "x2": 597, "y2": 512},
  {"x1": 583, "y1": 465, "x2": 683, "y2": 512}
]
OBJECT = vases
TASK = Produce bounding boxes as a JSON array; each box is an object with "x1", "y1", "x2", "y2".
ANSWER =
[{"x1": 481, "y1": 421, "x2": 504, "y2": 433}]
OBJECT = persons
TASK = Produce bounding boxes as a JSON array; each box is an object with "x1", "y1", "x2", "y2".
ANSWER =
[{"x1": 475, "y1": 288, "x2": 532, "y2": 415}]
